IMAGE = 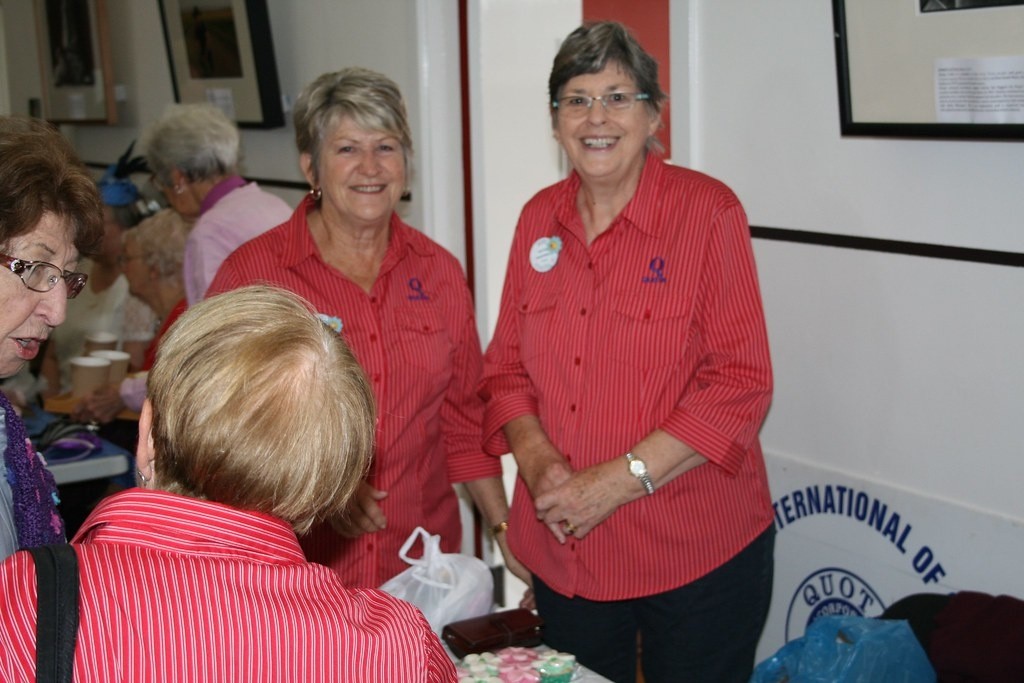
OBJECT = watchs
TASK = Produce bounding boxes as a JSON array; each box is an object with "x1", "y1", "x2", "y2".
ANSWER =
[{"x1": 625, "y1": 451, "x2": 654, "y2": 495}]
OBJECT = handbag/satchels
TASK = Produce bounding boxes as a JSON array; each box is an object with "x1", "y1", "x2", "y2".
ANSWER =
[
  {"x1": 376, "y1": 528, "x2": 494, "y2": 645},
  {"x1": 749, "y1": 615, "x2": 935, "y2": 683}
]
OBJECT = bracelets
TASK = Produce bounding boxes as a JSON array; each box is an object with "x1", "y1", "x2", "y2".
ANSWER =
[{"x1": 488, "y1": 521, "x2": 511, "y2": 534}]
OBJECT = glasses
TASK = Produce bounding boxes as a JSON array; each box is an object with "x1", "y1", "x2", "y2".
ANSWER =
[
  {"x1": 550, "y1": 92, "x2": 650, "y2": 118},
  {"x1": 149, "y1": 169, "x2": 165, "y2": 192},
  {"x1": 0, "y1": 253, "x2": 88, "y2": 299}
]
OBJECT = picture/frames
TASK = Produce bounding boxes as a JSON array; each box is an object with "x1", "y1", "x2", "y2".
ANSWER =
[
  {"x1": 157, "y1": 0, "x2": 286, "y2": 131},
  {"x1": 832, "y1": 1, "x2": 1024, "y2": 143},
  {"x1": 31, "y1": 0, "x2": 118, "y2": 127}
]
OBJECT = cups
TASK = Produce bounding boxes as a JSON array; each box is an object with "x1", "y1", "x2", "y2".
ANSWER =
[{"x1": 67, "y1": 329, "x2": 130, "y2": 400}]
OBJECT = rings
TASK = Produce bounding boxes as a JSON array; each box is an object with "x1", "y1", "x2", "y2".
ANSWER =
[{"x1": 567, "y1": 523, "x2": 576, "y2": 532}]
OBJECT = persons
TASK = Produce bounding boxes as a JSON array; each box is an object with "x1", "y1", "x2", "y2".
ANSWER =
[
  {"x1": 479, "y1": 20, "x2": 777, "y2": 683},
  {"x1": 0, "y1": 114, "x2": 103, "y2": 561},
  {"x1": 140, "y1": 101, "x2": 294, "y2": 306},
  {"x1": 0, "y1": 285, "x2": 459, "y2": 683},
  {"x1": 16, "y1": 174, "x2": 191, "y2": 426},
  {"x1": 204, "y1": 69, "x2": 536, "y2": 613}
]
